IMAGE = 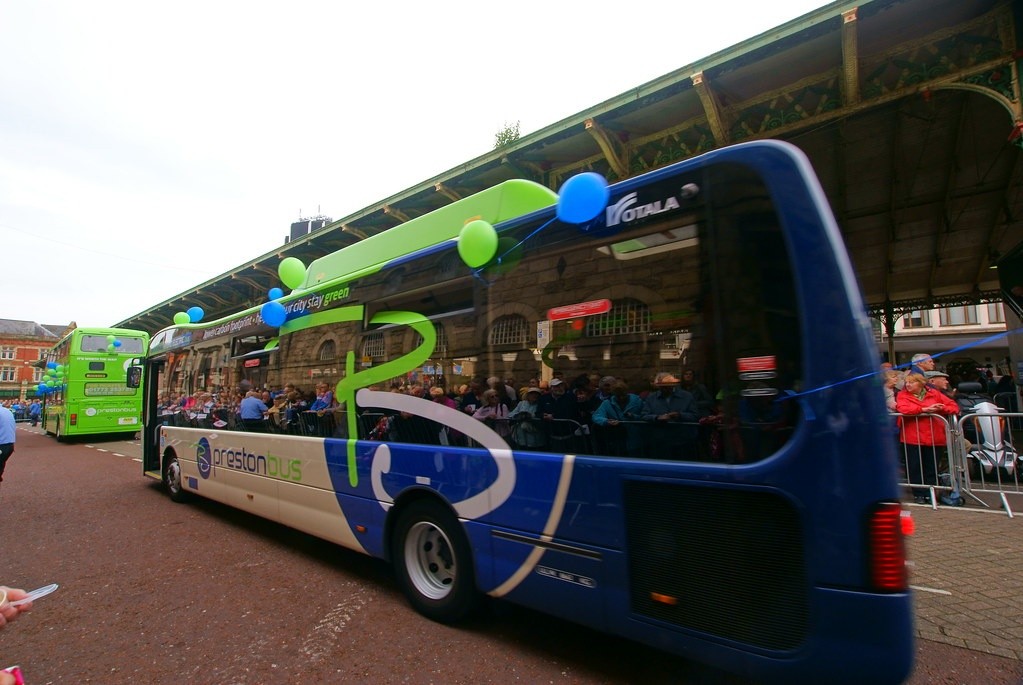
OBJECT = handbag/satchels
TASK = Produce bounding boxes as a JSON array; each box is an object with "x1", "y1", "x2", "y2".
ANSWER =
[{"x1": 606, "y1": 400, "x2": 626, "y2": 443}]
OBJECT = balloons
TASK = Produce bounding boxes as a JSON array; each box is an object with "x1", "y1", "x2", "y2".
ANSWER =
[
  {"x1": 187, "y1": 307, "x2": 204, "y2": 322},
  {"x1": 261, "y1": 301, "x2": 286, "y2": 327},
  {"x1": 556, "y1": 172, "x2": 608, "y2": 224},
  {"x1": 278, "y1": 257, "x2": 306, "y2": 289},
  {"x1": 174, "y1": 312, "x2": 190, "y2": 324},
  {"x1": 106, "y1": 335, "x2": 121, "y2": 351},
  {"x1": 457, "y1": 220, "x2": 498, "y2": 268},
  {"x1": 268, "y1": 288, "x2": 283, "y2": 300},
  {"x1": 32, "y1": 362, "x2": 64, "y2": 396}
]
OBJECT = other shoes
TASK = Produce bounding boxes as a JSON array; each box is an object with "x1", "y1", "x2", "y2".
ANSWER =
[
  {"x1": 913, "y1": 495, "x2": 925, "y2": 504},
  {"x1": 925, "y1": 497, "x2": 932, "y2": 504}
]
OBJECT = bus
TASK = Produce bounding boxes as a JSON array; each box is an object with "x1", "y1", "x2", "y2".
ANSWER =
[
  {"x1": 40, "y1": 328, "x2": 151, "y2": 442},
  {"x1": 123, "y1": 138, "x2": 918, "y2": 685}
]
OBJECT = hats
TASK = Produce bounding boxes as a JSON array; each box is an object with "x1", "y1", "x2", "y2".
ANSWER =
[
  {"x1": 548, "y1": 378, "x2": 562, "y2": 389},
  {"x1": 528, "y1": 387, "x2": 541, "y2": 395},
  {"x1": 655, "y1": 374, "x2": 681, "y2": 386},
  {"x1": 924, "y1": 371, "x2": 949, "y2": 379}
]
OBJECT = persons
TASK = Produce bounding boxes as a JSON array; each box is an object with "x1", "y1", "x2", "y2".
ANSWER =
[
  {"x1": 0, "y1": 399, "x2": 42, "y2": 426},
  {"x1": 158, "y1": 380, "x2": 345, "y2": 434},
  {"x1": 882, "y1": 353, "x2": 972, "y2": 503},
  {"x1": 0, "y1": 406, "x2": 16, "y2": 481},
  {"x1": 368, "y1": 371, "x2": 698, "y2": 441}
]
